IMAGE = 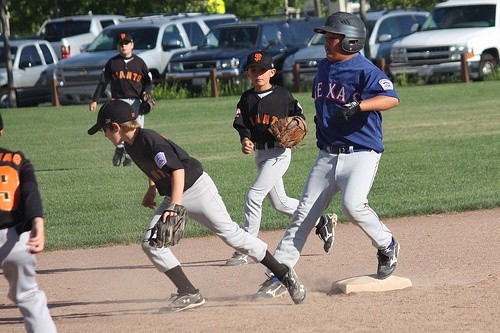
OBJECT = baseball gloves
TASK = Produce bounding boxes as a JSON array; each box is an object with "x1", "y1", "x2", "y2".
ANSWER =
[
  {"x1": 139, "y1": 98, "x2": 155, "y2": 116},
  {"x1": 268, "y1": 116, "x2": 308, "y2": 148},
  {"x1": 148, "y1": 204, "x2": 187, "y2": 248}
]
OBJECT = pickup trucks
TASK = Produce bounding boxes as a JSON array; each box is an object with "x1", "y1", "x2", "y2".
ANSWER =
[
  {"x1": 0, "y1": 11, "x2": 128, "y2": 108},
  {"x1": 56, "y1": 11, "x2": 242, "y2": 105},
  {"x1": 165, "y1": 10, "x2": 325, "y2": 95}
]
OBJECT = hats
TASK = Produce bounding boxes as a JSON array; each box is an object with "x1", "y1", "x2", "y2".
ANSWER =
[
  {"x1": 242, "y1": 51, "x2": 275, "y2": 69},
  {"x1": 117, "y1": 33, "x2": 134, "y2": 43},
  {"x1": 87, "y1": 99, "x2": 136, "y2": 135}
]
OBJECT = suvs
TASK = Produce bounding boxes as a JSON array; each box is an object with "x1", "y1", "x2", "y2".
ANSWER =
[{"x1": 283, "y1": 8, "x2": 430, "y2": 86}]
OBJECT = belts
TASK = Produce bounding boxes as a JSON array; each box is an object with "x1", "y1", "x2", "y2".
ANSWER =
[
  {"x1": 320, "y1": 146, "x2": 357, "y2": 153},
  {"x1": 254, "y1": 142, "x2": 275, "y2": 150}
]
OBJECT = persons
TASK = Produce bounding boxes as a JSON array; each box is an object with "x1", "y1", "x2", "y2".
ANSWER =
[
  {"x1": 87, "y1": 101, "x2": 306, "y2": 313},
  {"x1": 251, "y1": 11, "x2": 401, "y2": 300},
  {"x1": 0, "y1": 113, "x2": 58, "y2": 333},
  {"x1": 225, "y1": 48, "x2": 337, "y2": 265},
  {"x1": 90, "y1": 30, "x2": 154, "y2": 167}
]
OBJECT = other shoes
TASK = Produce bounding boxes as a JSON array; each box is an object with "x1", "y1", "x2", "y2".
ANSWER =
[
  {"x1": 122, "y1": 154, "x2": 133, "y2": 167},
  {"x1": 112, "y1": 147, "x2": 125, "y2": 166}
]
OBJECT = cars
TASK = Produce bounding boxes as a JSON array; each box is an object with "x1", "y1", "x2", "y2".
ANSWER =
[{"x1": 389, "y1": 0, "x2": 500, "y2": 82}]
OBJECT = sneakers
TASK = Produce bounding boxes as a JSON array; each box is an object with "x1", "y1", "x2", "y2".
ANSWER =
[
  {"x1": 376, "y1": 236, "x2": 400, "y2": 278},
  {"x1": 278, "y1": 263, "x2": 306, "y2": 304},
  {"x1": 226, "y1": 251, "x2": 259, "y2": 266},
  {"x1": 253, "y1": 278, "x2": 288, "y2": 303},
  {"x1": 158, "y1": 288, "x2": 206, "y2": 314},
  {"x1": 318, "y1": 213, "x2": 337, "y2": 253}
]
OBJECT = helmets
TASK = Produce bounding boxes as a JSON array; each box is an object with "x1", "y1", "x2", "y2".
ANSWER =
[{"x1": 313, "y1": 11, "x2": 366, "y2": 53}]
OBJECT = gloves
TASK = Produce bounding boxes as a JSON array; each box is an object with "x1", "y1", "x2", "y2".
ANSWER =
[{"x1": 335, "y1": 101, "x2": 359, "y2": 122}]
